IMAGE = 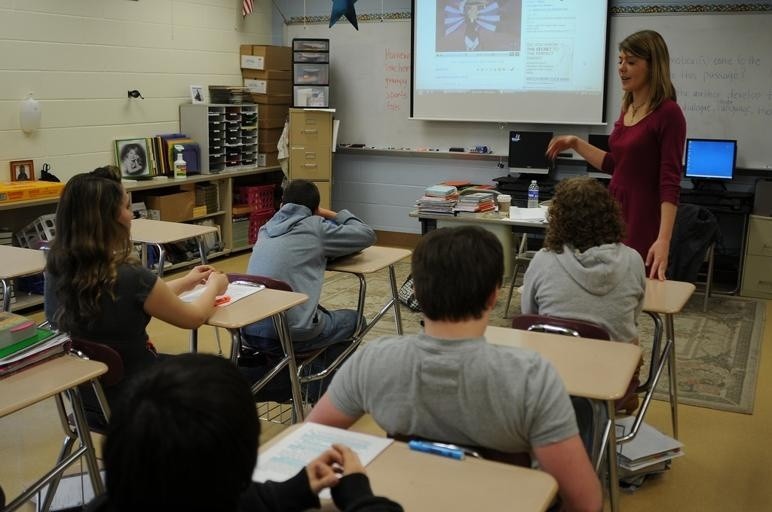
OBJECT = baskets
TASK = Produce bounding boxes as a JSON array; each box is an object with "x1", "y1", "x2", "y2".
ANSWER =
[
  {"x1": 236, "y1": 182, "x2": 276, "y2": 214},
  {"x1": 16, "y1": 213, "x2": 58, "y2": 252},
  {"x1": 248, "y1": 209, "x2": 276, "y2": 244}
]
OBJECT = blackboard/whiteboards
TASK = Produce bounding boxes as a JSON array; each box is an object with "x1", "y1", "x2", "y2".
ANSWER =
[{"x1": 282, "y1": 5, "x2": 772, "y2": 177}]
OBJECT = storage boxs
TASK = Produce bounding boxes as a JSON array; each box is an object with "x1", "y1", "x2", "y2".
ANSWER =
[
  {"x1": 239, "y1": 42, "x2": 293, "y2": 166},
  {"x1": 148, "y1": 189, "x2": 194, "y2": 223}
]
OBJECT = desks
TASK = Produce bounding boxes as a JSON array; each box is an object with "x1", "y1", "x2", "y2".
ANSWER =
[{"x1": 1, "y1": 199, "x2": 772, "y2": 511}]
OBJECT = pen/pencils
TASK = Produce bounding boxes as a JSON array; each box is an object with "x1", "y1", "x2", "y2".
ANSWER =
[
  {"x1": 216, "y1": 296, "x2": 231, "y2": 305},
  {"x1": 408, "y1": 439, "x2": 465, "y2": 461}
]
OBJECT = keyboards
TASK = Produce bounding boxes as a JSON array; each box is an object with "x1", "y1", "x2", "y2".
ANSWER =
[
  {"x1": 500, "y1": 182, "x2": 554, "y2": 193},
  {"x1": 680, "y1": 195, "x2": 735, "y2": 207}
]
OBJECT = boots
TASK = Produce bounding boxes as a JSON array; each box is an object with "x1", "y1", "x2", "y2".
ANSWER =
[{"x1": 618, "y1": 356, "x2": 643, "y2": 416}]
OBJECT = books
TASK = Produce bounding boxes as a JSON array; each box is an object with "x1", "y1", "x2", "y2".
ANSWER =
[{"x1": 415, "y1": 185, "x2": 496, "y2": 214}]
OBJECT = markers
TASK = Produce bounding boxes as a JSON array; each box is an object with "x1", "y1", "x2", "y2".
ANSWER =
[{"x1": 367, "y1": 146, "x2": 440, "y2": 151}]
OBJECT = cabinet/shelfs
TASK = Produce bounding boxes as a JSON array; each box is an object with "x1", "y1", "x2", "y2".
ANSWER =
[
  {"x1": 180, "y1": 103, "x2": 259, "y2": 173},
  {"x1": 292, "y1": 39, "x2": 330, "y2": 109},
  {"x1": 1, "y1": 167, "x2": 280, "y2": 314},
  {"x1": 288, "y1": 108, "x2": 332, "y2": 210}
]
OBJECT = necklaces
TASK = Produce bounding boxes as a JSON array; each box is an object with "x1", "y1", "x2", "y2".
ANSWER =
[{"x1": 629, "y1": 98, "x2": 647, "y2": 122}]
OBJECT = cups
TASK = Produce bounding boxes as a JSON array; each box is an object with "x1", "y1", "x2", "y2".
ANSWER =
[{"x1": 496, "y1": 194, "x2": 512, "y2": 218}]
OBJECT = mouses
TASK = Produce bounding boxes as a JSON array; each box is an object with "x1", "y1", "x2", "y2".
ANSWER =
[{"x1": 733, "y1": 199, "x2": 742, "y2": 210}]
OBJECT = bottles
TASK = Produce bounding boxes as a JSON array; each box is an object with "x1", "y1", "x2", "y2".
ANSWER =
[{"x1": 528, "y1": 179, "x2": 539, "y2": 209}]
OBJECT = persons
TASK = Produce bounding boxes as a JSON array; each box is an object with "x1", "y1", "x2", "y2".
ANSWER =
[
  {"x1": 43, "y1": 164, "x2": 229, "y2": 362},
  {"x1": 240, "y1": 178, "x2": 378, "y2": 352},
  {"x1": 18, "y1": 165, "x2": 28, "y2": 179},
  {"x1": 519, "y1": 175, "x2": 647, "y2": 346},
  {"x1": 545, "y1": 28, "x2": 688, "y2": 281},
  {"x1": 303, "y1": 223, "x2": 604, "y2": 512},
  {"x1": 42, "y1": 352, "x2": 405, "y2": 512},
  {"x1": 124, "y1": 146, "x2": 143, "y2": 174}
]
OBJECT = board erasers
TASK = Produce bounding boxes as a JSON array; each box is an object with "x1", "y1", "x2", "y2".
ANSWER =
[
  {"x1": 340, "y1": 143, "x2": 366, "y2": 149},
  {"x1": 449, "y1": 147, "x2": 464, "y2": 152}
]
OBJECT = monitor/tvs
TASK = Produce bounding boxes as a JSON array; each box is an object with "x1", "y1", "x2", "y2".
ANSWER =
[
  {"x1": 685, "y1": 138, "x2": 736, "y2": 191},
  {"x1": 509, "y1": 131, "x2": 553, "y2": 184},
  {"x1": 587, "y1": 135, "x2": 613, "y2": 179}
]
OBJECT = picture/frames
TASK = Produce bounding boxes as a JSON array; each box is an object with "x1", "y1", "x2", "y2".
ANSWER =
[
  {"x1": 10, "y1": 159, "x2": 35, "y2": 180},
  {"x1": 191, "y1": 86, "x2": 205, "y2": 105},
  {"x1": 114, "y1": 138, "x2": 152, "y2": 177}
]
